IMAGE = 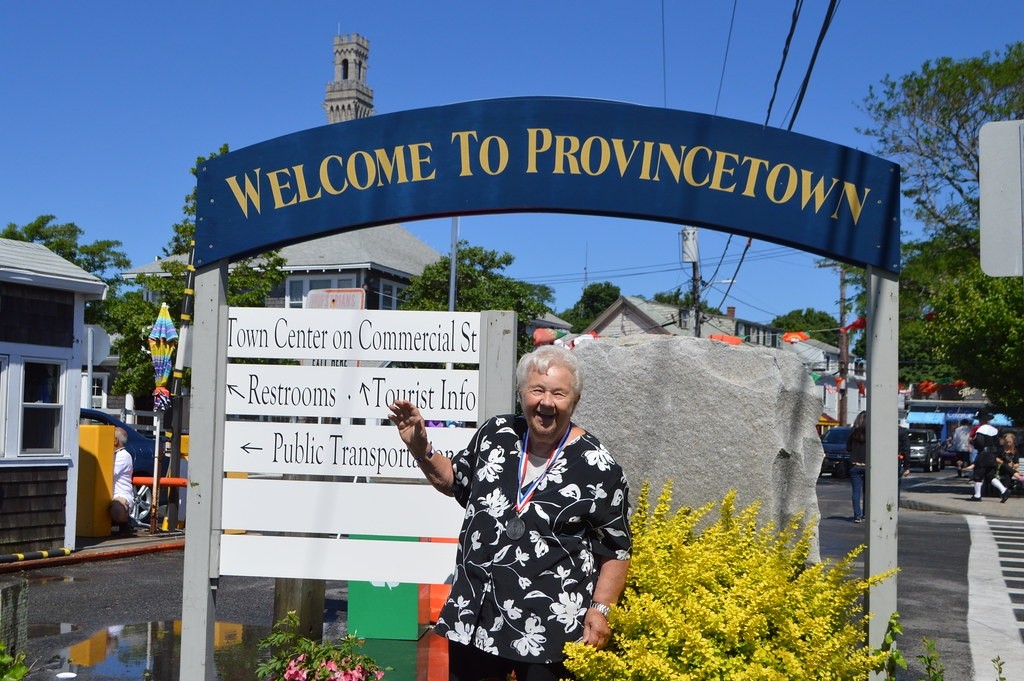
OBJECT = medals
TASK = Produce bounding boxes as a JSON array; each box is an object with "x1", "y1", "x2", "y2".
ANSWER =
[{"x1": 506, "y1": 516, "x2": 525, "y2": 540}]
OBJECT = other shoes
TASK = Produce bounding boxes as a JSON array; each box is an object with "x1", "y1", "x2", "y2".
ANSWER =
[
  {"x1": 119, "y1": 522, "x2": 134, "y2": 538},
  {"x1": 957, "y1": 471, "x2": 962, "y2": 477},
  {"x1": 1019, "y1": 476, "x2": 1024, "y2": 482}
]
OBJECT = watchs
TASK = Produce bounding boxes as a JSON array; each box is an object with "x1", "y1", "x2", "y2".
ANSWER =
[{"x1": 589, "y1": 602, "x2": 610, "y2": 620}]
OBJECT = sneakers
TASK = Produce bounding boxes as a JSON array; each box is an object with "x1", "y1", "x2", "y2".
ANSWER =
[
  {"x1": 966, "y1": 495, "x2": 981, "y2": 501},
  {"x1": 1000, "y1": 487, "x2": 1012, "y2": 503},
  {"x1": 854, "y1": 516, "x2": 866, "y2": 523}
]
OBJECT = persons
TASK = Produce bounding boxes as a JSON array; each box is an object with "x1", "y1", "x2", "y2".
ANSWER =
[
  {"x1": 387, "y1": 344, "x2": 634, "y2": 680},
  {"x1": 965, "y1": 407, "x2": 1011, "y2": 504},
  {"x1": 897, "y1": 426, "x2": 911, "y2": 509},
  {"x1": 995, "y1": 433, "x2": 1024, "y2": 499},
  {"x1": 952, "y1": 418, "x2": 980, "y2": 478},
  {"x1": 847, "y1": 410, "x2": 867, "y2": 523}
]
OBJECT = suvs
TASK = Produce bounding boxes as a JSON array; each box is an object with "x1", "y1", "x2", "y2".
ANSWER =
[
  {"x1": 906, "y1": 428, "x2": 942, "y2": 473},
  {"x1": 818, "y1": 427, "x2": 854, "y2": 478}
]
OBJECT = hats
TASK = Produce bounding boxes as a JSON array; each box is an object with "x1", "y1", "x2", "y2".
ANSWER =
[{"x1": 972, "y1": 411, "x2": 994, "y2": 421}]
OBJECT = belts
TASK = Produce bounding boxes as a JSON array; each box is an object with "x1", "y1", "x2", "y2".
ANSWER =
[{"x1": 852, "y1": 461, "x2": 866, "y2": 466}]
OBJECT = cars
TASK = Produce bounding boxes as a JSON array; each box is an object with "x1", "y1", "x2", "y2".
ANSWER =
[{"x1": 80, "y1": 408, "x2": 178, "y2": 524}]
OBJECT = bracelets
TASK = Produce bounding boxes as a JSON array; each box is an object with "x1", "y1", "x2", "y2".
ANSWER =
[{"x1": 416, "y1": 444, "x2": 435, "y2": 463}]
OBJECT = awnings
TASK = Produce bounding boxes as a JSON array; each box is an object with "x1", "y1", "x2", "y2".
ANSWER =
[
  {"x1": 906, "y1": 405, "x2": 945, "y2": 424},
  {"x1": 945, "y1": 408, "x2": 976, "y2": 422},
  {"x1": 973, "y1": 407, "x2": 1014, "y2": 427}
]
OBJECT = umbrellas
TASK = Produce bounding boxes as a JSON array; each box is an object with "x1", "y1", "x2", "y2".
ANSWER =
[{"x1": 149, "y1": 302, "x2": 177, "y2": 535}]
